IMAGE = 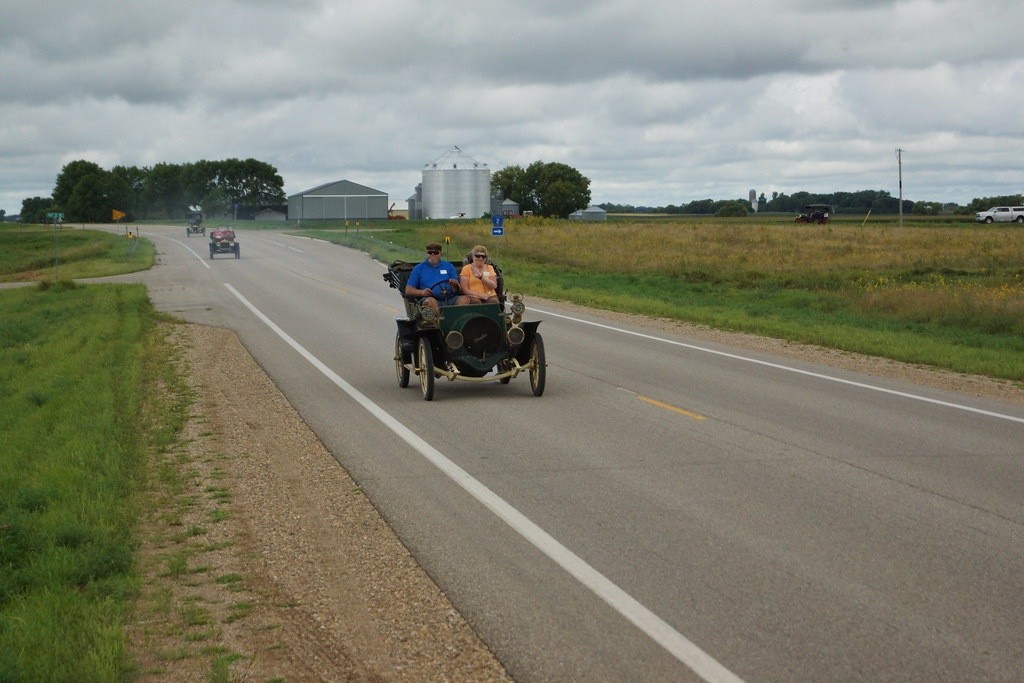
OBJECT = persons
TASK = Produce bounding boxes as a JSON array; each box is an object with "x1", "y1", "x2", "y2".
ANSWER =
[
  {"x1": 224, "y1": 227, "x2": 234, "y2": 241},
  {"x1": 213, "y1": 227, "x2": 224, "y2": 241},
  {"x1": 459, "y1": 246, "x2": 500, "y2": 305},
  {"x1": 405, "y1": 243, "x2": 470, "y2": 324}
]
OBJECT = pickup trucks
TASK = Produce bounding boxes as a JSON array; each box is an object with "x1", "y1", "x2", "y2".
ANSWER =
[{"x1": 974, "y1": 204, "x2": 1024, "y2": 225}]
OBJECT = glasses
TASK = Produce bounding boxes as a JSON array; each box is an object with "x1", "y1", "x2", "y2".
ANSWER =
[
  {"x1": 474, "y1": 255, "x2": 487, "y2": 259},
  {"x1": 427, "y1": 251, "x2": 439, "y2": 255}
]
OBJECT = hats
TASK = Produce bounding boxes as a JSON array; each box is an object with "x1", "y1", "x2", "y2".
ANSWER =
[{"x1": 426, "y1": 244, "x2": 442, "y2": 251}]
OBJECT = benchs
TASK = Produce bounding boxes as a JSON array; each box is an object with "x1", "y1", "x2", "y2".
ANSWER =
[
  {"x1": 398, "y1": 269, "x2": 504, "y2": 304},
  {"x1": 211, "y1": 231, "x2": 235, "y2": 239}
]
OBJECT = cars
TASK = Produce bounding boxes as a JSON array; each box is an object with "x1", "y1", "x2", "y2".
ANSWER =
[
  {"x1": 185, "y1": 212, "x2": 206, "y2": 238},
  {"x1": 208, "y1": 226, "x2": 240, "y2": 260},
  {"x1": 794, "y1": 203, "x2": 835, "y2": 226},
  {"x1": 381, "y1": 256, "x2": 546, "y2": 403}
]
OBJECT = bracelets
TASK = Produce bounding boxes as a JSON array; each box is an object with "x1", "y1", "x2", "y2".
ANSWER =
[{"x1": 479, "y1": 276, "x2": 482, "y2": 279}]
